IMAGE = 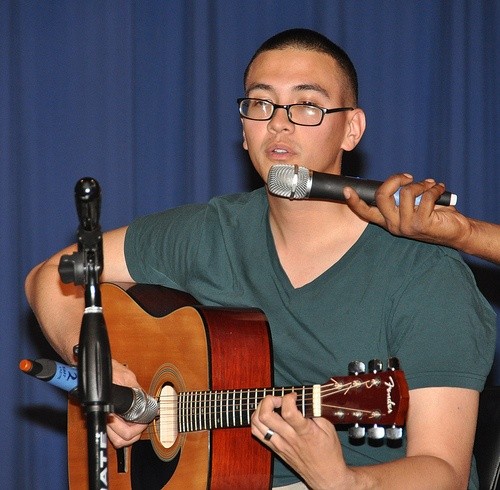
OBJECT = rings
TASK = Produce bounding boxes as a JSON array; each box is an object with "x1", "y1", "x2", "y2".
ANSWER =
[{"x1": 264, "y1": 429, "x2": 276, "y2": 441}]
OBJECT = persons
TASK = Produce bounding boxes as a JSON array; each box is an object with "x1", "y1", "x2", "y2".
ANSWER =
[
  {"x1": 24, "y1": 26, "x2": 500, "y2": 490},
  {"x1": 342, "y1": 171, "x2": 499, "y2": 267}
]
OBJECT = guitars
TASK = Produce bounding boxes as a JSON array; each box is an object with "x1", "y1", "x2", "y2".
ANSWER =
[{"x1": 67, "y1": 280, "x2": 410, "y2": 490}]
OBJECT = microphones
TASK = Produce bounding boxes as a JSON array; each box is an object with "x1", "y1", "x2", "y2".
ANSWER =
[
  {"x1": 20, "y1": 359, "x2": 158, "y2": 424},
  {"x1": 266, "y1": 164, "x2": 456, "y2": 208}
]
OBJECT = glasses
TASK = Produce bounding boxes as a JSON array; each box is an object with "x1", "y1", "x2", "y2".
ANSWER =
[{"x1": 236, "y1": 97, "x2": 354, "y2": 126}]
